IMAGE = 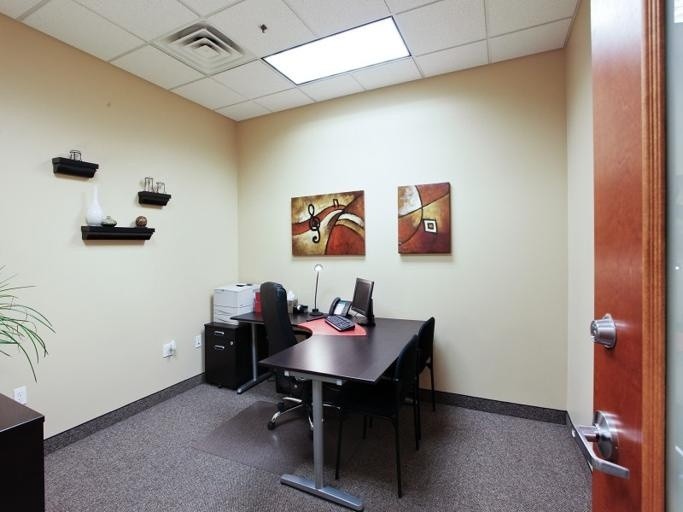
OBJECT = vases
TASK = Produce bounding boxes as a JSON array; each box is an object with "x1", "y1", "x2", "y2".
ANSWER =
[{"x1": 84, "y1": 185, "x2": 103, "y2": 227}]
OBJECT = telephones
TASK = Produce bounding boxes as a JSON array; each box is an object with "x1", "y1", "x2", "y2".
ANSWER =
[{"x1": 329, "y1": 297, "x2": 352, "y2": 317}]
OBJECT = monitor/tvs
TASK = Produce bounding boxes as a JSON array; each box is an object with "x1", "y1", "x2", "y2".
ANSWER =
[{"x1": 351, "y1": 277, "x2": 375, "y2": 328}]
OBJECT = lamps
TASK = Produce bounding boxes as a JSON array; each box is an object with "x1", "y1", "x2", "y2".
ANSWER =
[{"x1": 310, "y1": 264, "x2": 323, "y2": 316}]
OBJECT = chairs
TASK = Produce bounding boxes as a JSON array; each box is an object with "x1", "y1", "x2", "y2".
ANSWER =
[
  {"x1": 335, "y1": 336, "x2": 420, "y2": 498},
  {"x1": 369, "y1": 317, "x2": 436, "y2": 440},
  {"x1": 260, "y1": 282, "x2": 341, "y2": 435}
]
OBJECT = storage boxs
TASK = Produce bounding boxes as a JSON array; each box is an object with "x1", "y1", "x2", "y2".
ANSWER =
[{"x1": 212, "y1": 284, "x2": 260, "y2": 325}]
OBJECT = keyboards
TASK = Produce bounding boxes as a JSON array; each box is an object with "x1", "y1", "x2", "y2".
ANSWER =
[{"x1": 325, "y1": 315, "x2": 355, "y2": 332}]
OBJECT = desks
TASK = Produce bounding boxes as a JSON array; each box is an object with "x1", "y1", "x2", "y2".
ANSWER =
[
  {"x1": 0, "y1": 393, "x2": 45, "y2": 511},
  {"x1": 230, "y1": 310, "x2": 427, "y2": 511}
]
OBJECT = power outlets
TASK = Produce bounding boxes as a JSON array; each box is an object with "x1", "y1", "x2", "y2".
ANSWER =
[{"x1": 13, "y1": 386, "x2": 27, "y2": 404}]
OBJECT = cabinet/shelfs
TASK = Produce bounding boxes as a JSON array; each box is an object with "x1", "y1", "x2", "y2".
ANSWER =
[{"x1": 204, "y1": 322, "x2": 267, "y2": 390}]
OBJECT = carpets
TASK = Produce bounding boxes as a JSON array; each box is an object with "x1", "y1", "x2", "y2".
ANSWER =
[{"x1": 192, "y1": 400, "x2": 314, "y2": 475}]
